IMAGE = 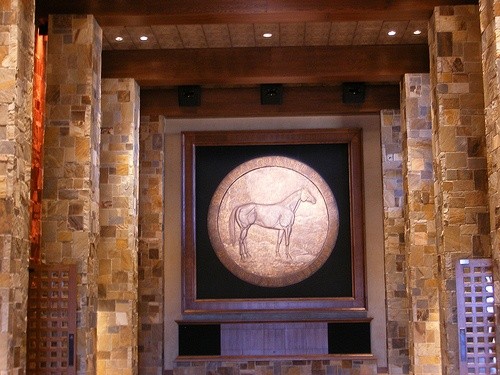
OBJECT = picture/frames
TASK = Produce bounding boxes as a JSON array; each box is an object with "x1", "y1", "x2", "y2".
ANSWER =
[{"x1": 179, "y1": 125, "x2": 369, "y2": 315}]
